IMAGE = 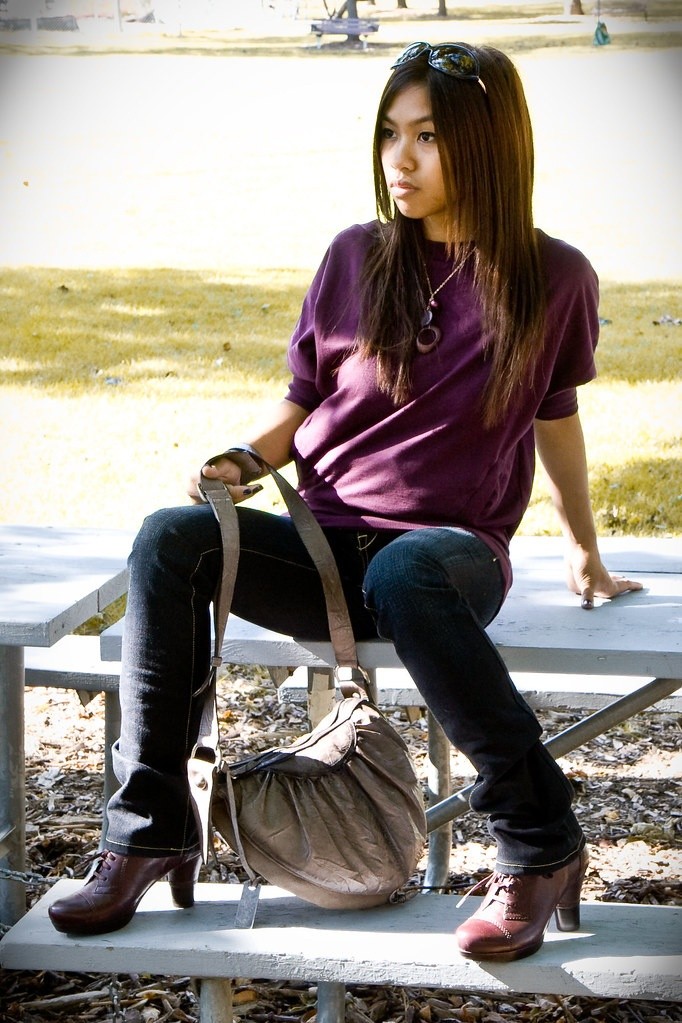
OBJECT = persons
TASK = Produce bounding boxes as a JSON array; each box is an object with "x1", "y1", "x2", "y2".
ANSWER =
[{"x1": 46, "y1": 38, "x2": 642, "y2": 963}]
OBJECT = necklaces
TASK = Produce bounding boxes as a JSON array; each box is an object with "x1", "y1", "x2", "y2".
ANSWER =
[{"x1": 414, "y1": 226, "x2": 479, "y2": 354}]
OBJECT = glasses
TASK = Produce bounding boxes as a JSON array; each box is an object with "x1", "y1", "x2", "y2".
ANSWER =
[{"x1": 390, "y1": 42, "x2": 486, "y2": 94}]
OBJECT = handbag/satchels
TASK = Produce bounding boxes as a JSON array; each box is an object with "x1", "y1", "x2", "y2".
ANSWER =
[{"x1": 185, "y1": 449, "x2": 427, "y2": 910}]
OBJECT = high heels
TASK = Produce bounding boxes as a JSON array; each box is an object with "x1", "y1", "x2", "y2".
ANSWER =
[
  {"x1": 455, "y1": 844, "x2": 589, "y2": 962},
  {"x1": 48, "y1": 849, "x2": 201, "y2": 936}
]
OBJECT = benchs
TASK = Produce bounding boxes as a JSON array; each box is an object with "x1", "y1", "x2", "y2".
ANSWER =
[
  {"x1": 277, "y1": 663, "x2": 682, "y2": 717},
  {"x1": 23, "y1": 634, "x2": 119, "y2": 696},
  {"x1": 0, "y1": 877, "x2": 682, "y2": 1001}
]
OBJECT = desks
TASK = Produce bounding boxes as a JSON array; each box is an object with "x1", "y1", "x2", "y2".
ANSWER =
[{"x1": 0, "y1": 518, "x2": 682, "y2": 1023}]
